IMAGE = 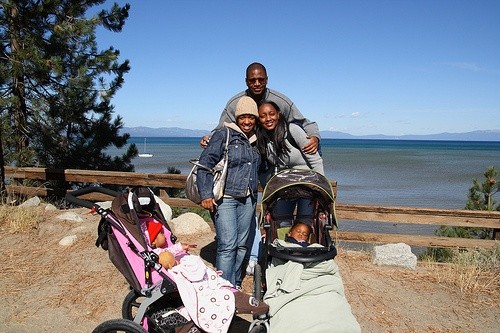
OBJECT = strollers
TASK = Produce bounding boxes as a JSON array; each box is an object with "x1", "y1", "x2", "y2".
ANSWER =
[
  {"x1": 65, "y1": 183, "x2": 271, "y2": 333},
  {"x1": 252, "y1": 167, "x2": 338, "y2": 312}
]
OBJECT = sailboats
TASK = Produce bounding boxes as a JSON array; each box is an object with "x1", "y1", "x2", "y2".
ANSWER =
[{"x1": 138, "y1": 138, "x2": 153, "y2": 158}]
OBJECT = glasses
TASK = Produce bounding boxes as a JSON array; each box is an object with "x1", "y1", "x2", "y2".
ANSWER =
[{"x1": 246, "y1": 77, "x2": 266, "y2": 84}]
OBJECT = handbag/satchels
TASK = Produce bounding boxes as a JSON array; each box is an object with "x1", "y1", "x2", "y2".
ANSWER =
[{"x1": 184, "y1": 128, "x2": 229, "y2": 204}]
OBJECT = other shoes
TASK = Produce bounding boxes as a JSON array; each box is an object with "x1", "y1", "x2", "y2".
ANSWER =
[{"x1": 245, "y1": 259, "x2": 256, "y2": 275}]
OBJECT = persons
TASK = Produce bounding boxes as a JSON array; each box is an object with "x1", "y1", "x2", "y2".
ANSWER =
[
  {"x1": 158, "y1": 250, "x2": 191, "y2": 269},
  {"x1": 139, "y1": 220, "x2": 270, "y2": 314},
  {"x1": 200, "y1": 63, "x2": 321, "y2": 155},
  {"x1": 197, "y1": 95, "x2": 263, "y2": 290},
  {"x1": 272, "y1": 222, "x2": 313, "y2": 266},
  {"x1": 255, "y1": 100, "x2": 325, "y2": 220}
]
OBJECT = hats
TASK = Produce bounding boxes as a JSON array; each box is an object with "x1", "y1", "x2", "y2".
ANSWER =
[
  {"x1": 145, "y1": 221, "x2": 162, "y2": 244},
  {"x1": 234, "y1": 96, "x2": 259, "y2": 118}
]
